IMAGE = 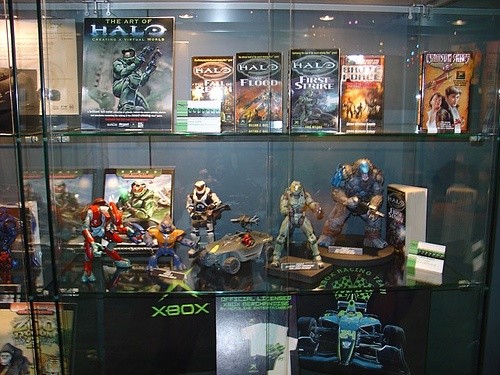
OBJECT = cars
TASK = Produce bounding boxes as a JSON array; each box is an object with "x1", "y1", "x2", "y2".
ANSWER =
[{"x1": 199, "y1": 214, "x2": 276, "y2": 275}]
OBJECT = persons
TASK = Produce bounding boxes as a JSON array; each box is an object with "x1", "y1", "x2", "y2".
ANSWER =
[
  {"x1": 318, "y1": 157, "x2": 390, "y2": 251},
  {"x1": 143, "y1": 213, "x2": 196, "y2": 272},
  {"x1": 427, "y1": 92, "x2": 445, "y2": 133},
  {"x1": 270, "y1": 181, "x2": 324, "y2": 268},
  {"x1": 186, "y1": 181, "x2": 226, "y2": 257},
  {"x1": 80, "y1": 198, "x2": 133, "y2": 282},
  {"x1": 436, "y1": 85, "x2": 462, "y2": 134}
]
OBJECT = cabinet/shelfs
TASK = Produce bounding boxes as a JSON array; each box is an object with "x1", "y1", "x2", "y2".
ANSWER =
[{"x1": 0, "y1": 0, "x2": 500, "y2": 375}]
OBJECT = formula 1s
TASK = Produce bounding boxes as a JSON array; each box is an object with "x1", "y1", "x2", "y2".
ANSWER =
[{"x1": 297, "y1": 293, "x2": 405, "y2": 375}]
